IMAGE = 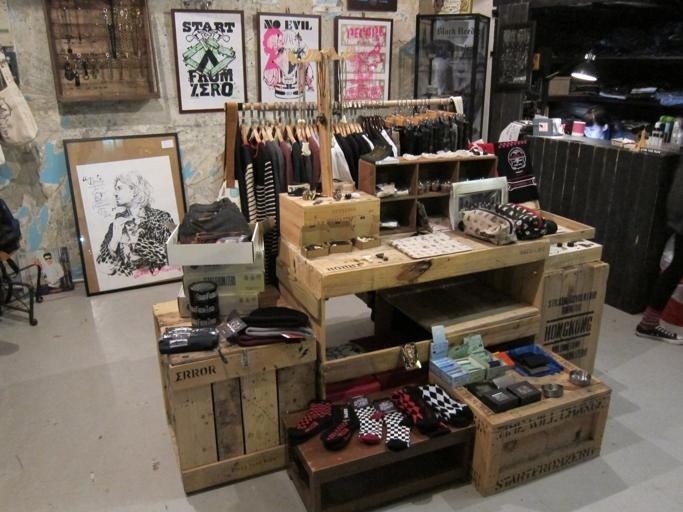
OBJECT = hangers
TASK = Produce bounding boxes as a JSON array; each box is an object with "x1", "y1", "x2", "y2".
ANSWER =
[{"x1": 238, "y1": 97, "x2": 460, "y2": 147}]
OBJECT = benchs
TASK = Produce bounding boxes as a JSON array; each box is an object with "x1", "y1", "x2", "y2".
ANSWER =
[{"x1": 281, "y1": 381, "x2": 477, "y2": 512}]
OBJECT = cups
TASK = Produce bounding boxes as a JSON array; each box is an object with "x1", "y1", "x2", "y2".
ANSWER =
[
  {"x1": 189, "y1": 281, "x2": 219, "y2": 327},
  {"x1": 572, "y1": 120, "x2": 586, "y2": 136}
]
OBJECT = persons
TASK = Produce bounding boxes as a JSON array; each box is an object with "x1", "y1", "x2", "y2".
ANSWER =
[
  {"x1": 41, "y1": 252, "x2": 65, "y2": 289},
  {"x1": 96, "y1": 170, "x2": 177, "y2": 278},
  {"x1": 635, "y1": 163, "x2": 683, "y2": 345}
]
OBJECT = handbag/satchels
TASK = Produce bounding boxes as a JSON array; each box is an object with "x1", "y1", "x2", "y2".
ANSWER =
[
  {"x1": 462, "y1": 204, "x2": 546, "y2": 246},
  {"x1": 0, "y1": 51, "x2": 39, "y2": 145},
  {"x1": 178, "y1": 197, "x2": 252, "y2": 244}
]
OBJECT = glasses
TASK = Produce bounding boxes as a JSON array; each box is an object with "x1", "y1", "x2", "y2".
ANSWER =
[{"x1": 45, "y1": 257, "x2": 52, "y2": 260}]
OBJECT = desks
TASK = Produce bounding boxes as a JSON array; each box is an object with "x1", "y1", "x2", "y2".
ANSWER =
[{"x1": 519, "y1": 136, "x2": 683, "y2": 315}]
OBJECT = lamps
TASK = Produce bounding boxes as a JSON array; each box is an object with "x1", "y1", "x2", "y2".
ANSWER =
[{"x1": 538, "y1": 46, "x2": 603, "y2": 110}]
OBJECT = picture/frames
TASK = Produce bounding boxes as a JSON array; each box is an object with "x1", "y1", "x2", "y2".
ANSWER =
[
  {"x1": 257, "y1": 12, "x2": 321, "y2": 102},
  {"x1": 335, "y1": 16, "x2": 392, "y2": 100},
  {"x1": 491, "y1": 22, "x2": 536, "y2": 92},
  {"x1": 348, "y1": 0, "x2": 397, "y2": 11},
  {"x1": 41, "y1": 0, "x2": 161, "y2": 107},
  {"x1": 172, "y1": 9, "x2": 247, "y2": 114},
  {"x1": 63, "y1": 132, "x2": 187, "y2": 297}
]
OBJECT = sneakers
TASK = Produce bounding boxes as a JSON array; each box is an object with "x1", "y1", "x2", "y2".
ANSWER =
[{"x1": 636, "y1": 323, "x2": 683, "y2": 344}]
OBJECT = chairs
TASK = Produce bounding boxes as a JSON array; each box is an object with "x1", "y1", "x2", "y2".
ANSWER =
[{"x1": 0, "y1": 256, "x2": 43, "y2": 325}]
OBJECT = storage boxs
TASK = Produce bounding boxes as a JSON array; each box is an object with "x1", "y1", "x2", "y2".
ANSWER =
[
  {"x1": 428, "y1": 344, "x2": 612, "y2": 497},
  {"x1": 153, "y1": 285, "x2": 317, "y2": 495}
]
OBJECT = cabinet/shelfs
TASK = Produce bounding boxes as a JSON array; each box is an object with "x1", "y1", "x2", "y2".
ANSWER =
[
  {"x1": 275, "y1": 152, "x2": 605, "y2": 403},
  {"x1": 415, "y1": 14, "x2": 488, "y2": 146},
  {"x1": 490, "y1": 1, "x2": 683, "y2": 128}
]
